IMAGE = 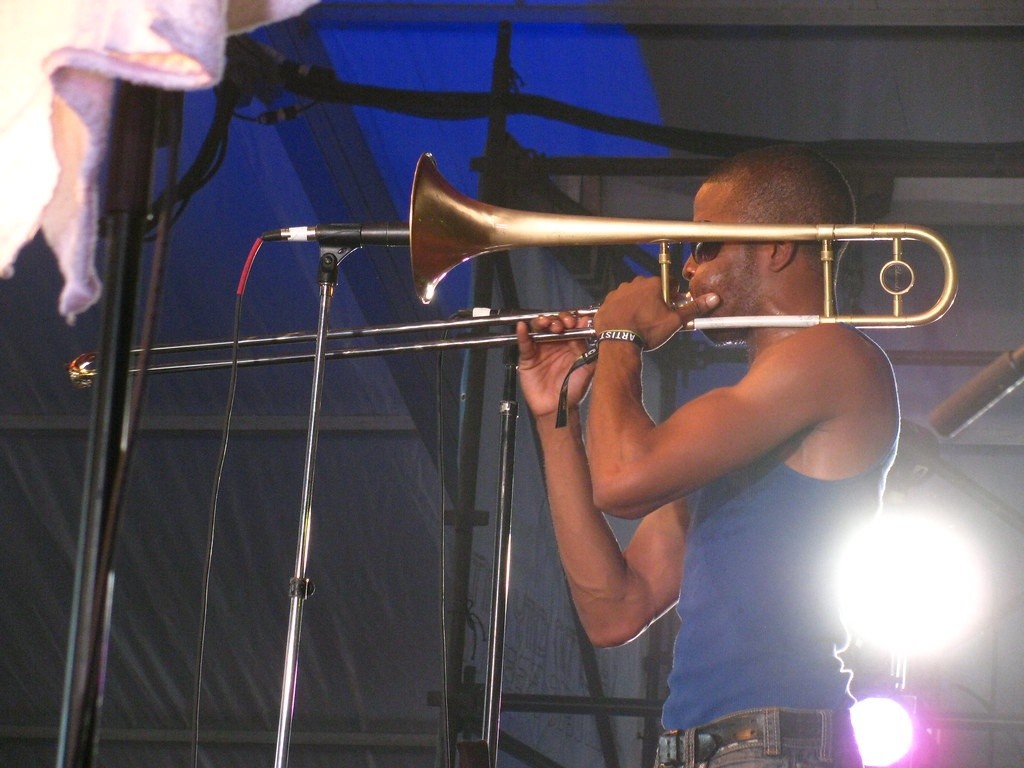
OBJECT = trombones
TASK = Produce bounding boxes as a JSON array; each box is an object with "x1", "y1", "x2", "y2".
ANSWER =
[{"x1": 68, "y1": 151, "x2": 960, "y2": 390}]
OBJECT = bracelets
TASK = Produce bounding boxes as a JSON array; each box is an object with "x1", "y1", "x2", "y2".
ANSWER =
[{"x1": 556, "y1": 330, "x2": 645, "y2": 427}]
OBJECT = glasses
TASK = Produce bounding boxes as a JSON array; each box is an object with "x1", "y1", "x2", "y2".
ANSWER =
[{"x1": 691, "y1": 219, "x2": 724, "y2": 264}]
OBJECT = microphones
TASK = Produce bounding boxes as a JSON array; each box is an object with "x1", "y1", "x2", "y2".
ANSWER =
[{"x1": 261, "y1": 221, "x2": 411, "y2": 248}]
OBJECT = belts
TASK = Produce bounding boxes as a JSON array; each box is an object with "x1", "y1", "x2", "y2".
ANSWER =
[{"x1": 658, "y1": 709, "x2": 855, "y2": 765}]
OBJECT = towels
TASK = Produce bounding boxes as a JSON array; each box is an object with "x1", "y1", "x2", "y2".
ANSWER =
[{"x1": 0, "y1": 0, "x2": 326, "y2": 325}]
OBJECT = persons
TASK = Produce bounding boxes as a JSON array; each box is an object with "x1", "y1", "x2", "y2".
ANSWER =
[{"x1": 517, "y1": 145, "x2": 901, "y2": 768}]
